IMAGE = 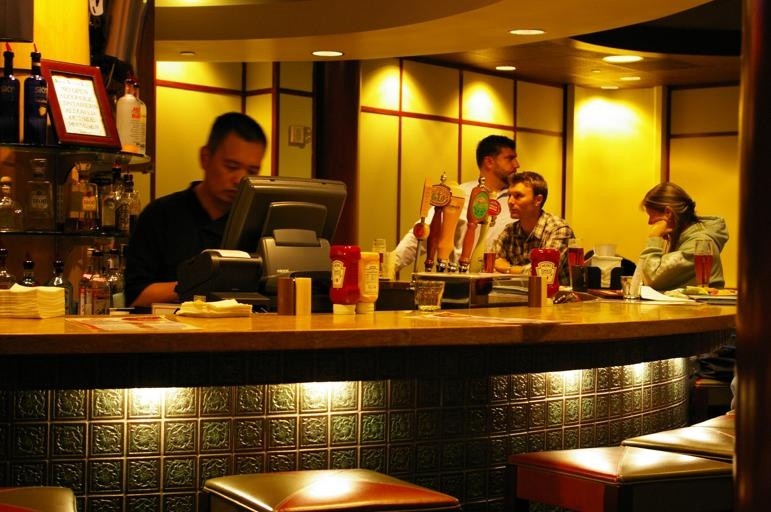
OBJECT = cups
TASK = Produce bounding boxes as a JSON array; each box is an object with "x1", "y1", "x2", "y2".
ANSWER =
[
  {"x1": 620, "y1": 274, "x2": 641, "y2": 301},
  {"x1": 480, "y1": 246, "x2": 498, "y2": 272},
  {"x1": 594, "y1": 242, "x2": 619, "y2": 257},
  {"x1": 693, "y1": 239, "x2": 714, "y2": 288},
  {"x1": 413, "y1": 280, "x2": 444, "y2": 312},
  {"x1": 567, "y1": 237, "x2": 590, "y2": 292}
]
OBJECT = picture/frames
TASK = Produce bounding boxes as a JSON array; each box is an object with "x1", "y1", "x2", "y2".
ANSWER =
[{"x1": 38, "y1": 58, "x2": 122, "y2": 151}]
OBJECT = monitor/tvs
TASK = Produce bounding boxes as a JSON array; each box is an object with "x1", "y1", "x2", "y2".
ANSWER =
[{"x1": 220, "y1": 175, "x2": 347, "y2": 256}]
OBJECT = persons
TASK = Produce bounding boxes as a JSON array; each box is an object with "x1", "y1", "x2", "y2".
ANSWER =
[
  {"x1": 486, "y1": 168, "x2": 578, "y2": 279},
  {"x1": 387, "y1": 133, "x2": 519, "y2": 271},
  {"x1": 120, "y1": 108, "x2": 269, "y2": 312},
  {"x1": 636, "y1": 180, "x2": 730, "y2": 294}
]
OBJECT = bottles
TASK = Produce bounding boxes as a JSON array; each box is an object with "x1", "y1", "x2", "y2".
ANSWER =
[
  {"x1": 529, "y1": 248, "x2": 561, "y2": 307},
  {"x1": 0, "y1": 36, "x2": 146, "y2": 320},
  {"x1": 328, "y1": 244, "x2": 380, "y2": 315}
]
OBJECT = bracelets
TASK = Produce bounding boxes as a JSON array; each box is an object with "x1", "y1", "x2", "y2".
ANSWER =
[{"x1": 504, "y1": 263, "x2": 515, "y2": 274}]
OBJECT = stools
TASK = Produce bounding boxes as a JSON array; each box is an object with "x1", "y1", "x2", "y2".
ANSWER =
[
  {"x1": 201, "y1": 465, "x2": 459, "y2": 511},
  {"x1": 506, "y1": 376, "x2": 737, "y2": 512},
  {"x1": 0, "y1": 482, "x2": 78, "y2": 512}
]
